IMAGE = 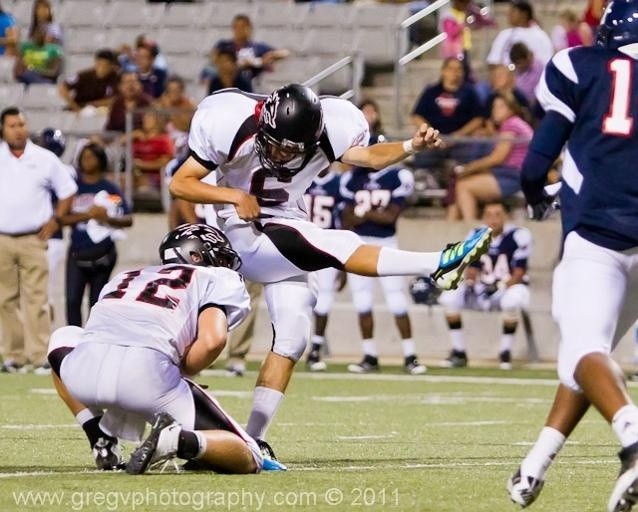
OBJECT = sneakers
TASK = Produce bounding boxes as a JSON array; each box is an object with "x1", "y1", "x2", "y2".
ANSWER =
[
  {"x1": 438, "y1": 350, "x2": 469, "y2": 369},
  {"x1": 0, "y1": 358, "x2": 27, "y2": 376},
  {"x1": 607, "y1": 442, "x2": 638, "y2": 512},
  {"x1": 127, "y1": 407, "x2": 184, "y2": 477},
  {"x1": 505, "y1": 463, "x2": 543, "y2": 510},
  {"x1": 347, "y1": 356, "x2": 380, "y2": 374},
  {"x1": 250, "y1": 439, "x2": 289, "y2": 473},
  {"x1": 31, "y1": 362, "x2": 53, "y2": 376},
  {"x1": 497, "y1": 348, "x2": 517, "y2": 371},
  {"x1": 304, "y1": 351, "x2": 327, "y2": 372},
  {"x1": 403, "y1": 355, "x2": 429, "y2": 376},
  {"x1": 429, "y1": 223, "x2": 493, "y2": 292},
  {"x1": 90, "y1": 437, "x2": 125, "y2": 470}
]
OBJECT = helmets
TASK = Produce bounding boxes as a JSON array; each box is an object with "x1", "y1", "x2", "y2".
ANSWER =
[
  {"x1": 595, "y1": 0, "x2": 638, "y2": 50},
  {"x1": 255, "y1": 81, "x2": 327, "y2": 154},
  {"x1": 159, "y1": 223, "x2": 236, "y2": 270}
]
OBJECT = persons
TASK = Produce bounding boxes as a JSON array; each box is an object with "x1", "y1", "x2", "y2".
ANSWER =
[{"x1": 1, "y1": 2, "x2": 638, "y2": 511}]
